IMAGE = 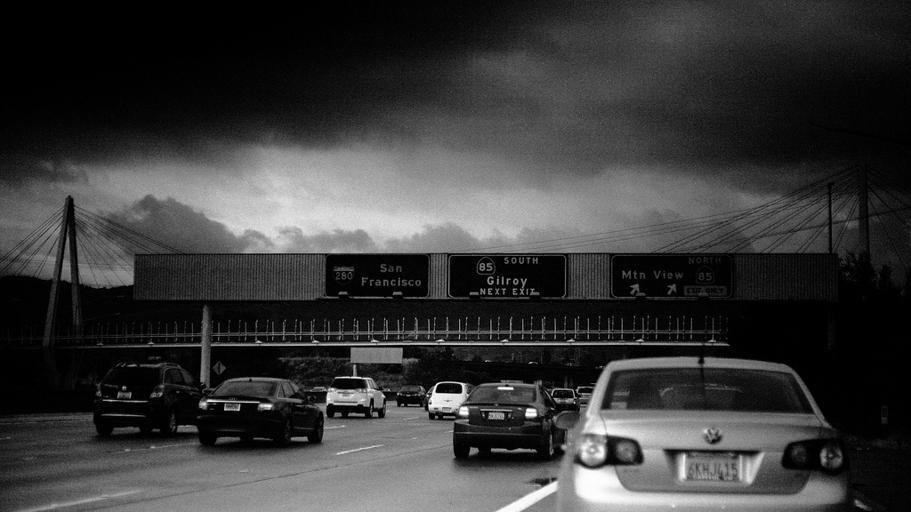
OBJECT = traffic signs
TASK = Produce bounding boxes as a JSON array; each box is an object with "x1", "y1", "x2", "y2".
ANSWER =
[
  {"x1": 324, "y1": 254, "x2": 430, "y2": 298},
  {"x1": 610, "y1": 252, "x2": 732, "y2": 298},
  {"x1": 448, "y1": 255, "x2": 566, "y2": 300}
]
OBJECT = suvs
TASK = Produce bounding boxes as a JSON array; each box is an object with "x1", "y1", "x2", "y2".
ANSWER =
[{"x1": 93, "y1": 362, "x2": 216, "y2": 439}]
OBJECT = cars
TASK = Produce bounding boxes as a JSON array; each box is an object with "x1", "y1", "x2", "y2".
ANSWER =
[
  {"x1": 655, "y1": 385, "x2": 743, "y2": 408},
  {"x1": 453, "y1": 379, "x2": 564, "y2": 465},
  {"x1": 196, "y1": 377, "x2": 324, "y2": 448},
  {"x1": 554, "y1": 352, "x2": 850, "y2": 510}
]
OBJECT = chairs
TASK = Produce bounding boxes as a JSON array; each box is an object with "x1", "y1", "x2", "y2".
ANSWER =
[
  {"x1": 627, "y1": 382, "x2": 664, "y2": 408},
  {"x1": 729, "y1": 373, "x2": 805, "y2": 413}
]
